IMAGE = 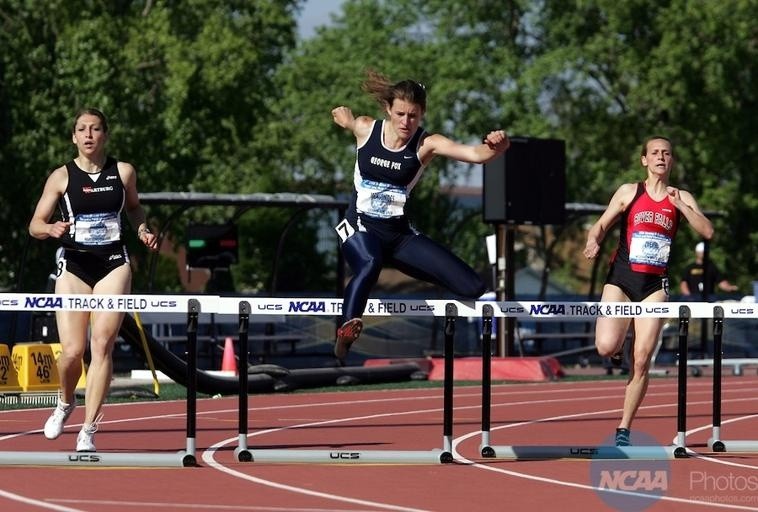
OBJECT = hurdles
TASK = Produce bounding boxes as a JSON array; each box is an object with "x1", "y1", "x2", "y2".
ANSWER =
[
  {"x1": 708, "y1": 300, "x2": 754, "y2": 456},
  {"x1": 220, "y1": 296, "x2": 474, "y2": 463},
  {"x1": 0, "y1": 294, "x2": 219, "y2": 466},
  {"x1": 474, "y1": 303, "x2": 708, "y2": 459}
]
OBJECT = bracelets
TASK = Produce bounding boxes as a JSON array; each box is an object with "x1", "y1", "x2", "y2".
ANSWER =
[{"x1": 137, "y1": 228, "x2": 152, "y2": 234}]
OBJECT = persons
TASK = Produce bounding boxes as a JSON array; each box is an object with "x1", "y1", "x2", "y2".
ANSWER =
[
  {"x1": 583, "y1": 137, "x2": 713, "y2": 447},
  {"x1": 331, "y1": 71, "x2": 510, "y2": 359},
  {"x1": 678, "y1": 240, "x2": 741, "y2": 296},
  {"x1": 29, "y1": 106, "x2": 159, "y2": 450}
]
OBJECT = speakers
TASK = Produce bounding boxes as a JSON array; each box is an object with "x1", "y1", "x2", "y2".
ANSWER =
[{"x1": 481, "y1": 136, "x2": 568, "y2": 226}]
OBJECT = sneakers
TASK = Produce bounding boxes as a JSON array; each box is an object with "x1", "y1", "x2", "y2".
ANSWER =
[
  {"x1": 75, "y1": 424, "x2": 100, "y2": 453},
  {"x1": 610, "y1": 427, "x2": 638, "y2": 447},
  {"x1": 43, "y1": 399, "x2": 73, "y2": 439},
  {"x1": 331, "y1": 318, "x2": 367, "y2": 358},
  {"x1": 608, "y1": 343, "x2": 626, "y2": 361}
]
